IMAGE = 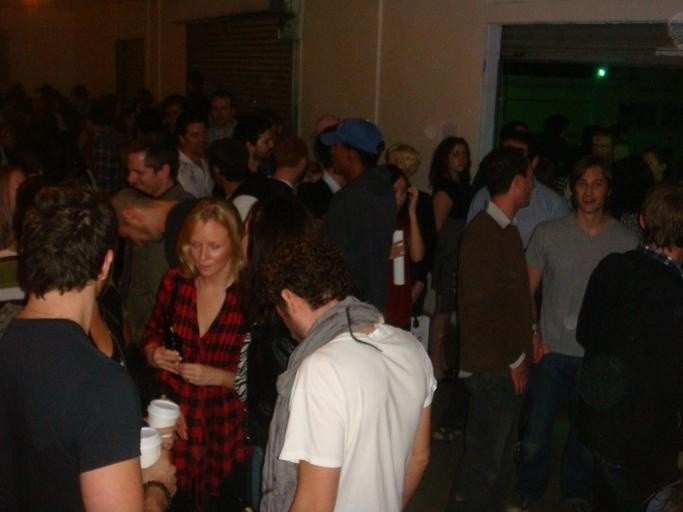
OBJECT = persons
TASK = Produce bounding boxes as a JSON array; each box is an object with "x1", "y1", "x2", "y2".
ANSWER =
[{"x1": 1, "y1": 81, "x2": 682, "y2": 511}]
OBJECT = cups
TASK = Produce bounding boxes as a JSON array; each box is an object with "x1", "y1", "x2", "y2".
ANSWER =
[
  {"x1": 146, "y1": 399, "x2": 181, "y2": 448},
  {"x1": 139, "y1": 427, "x2": 161, "y2": 471}
]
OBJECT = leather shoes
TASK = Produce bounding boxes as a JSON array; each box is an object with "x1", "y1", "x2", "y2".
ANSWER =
[
  {"x1": 444, "y1": 368, "x2": 459, "y2": 376},
  {"x1": 439, "y1": 377, "x2": 455, "y2": 385}
]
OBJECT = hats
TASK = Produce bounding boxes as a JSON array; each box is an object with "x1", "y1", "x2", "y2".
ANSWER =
[
  {"x1": 271, "y1": 132, "x2": 305, "y2": 166},
  {"x1": 312, "y1": 117, "x2": 340, "y2": 144},
  {"x1": 320, "y1": 118, "x2": 384, "y2": 155}
]
